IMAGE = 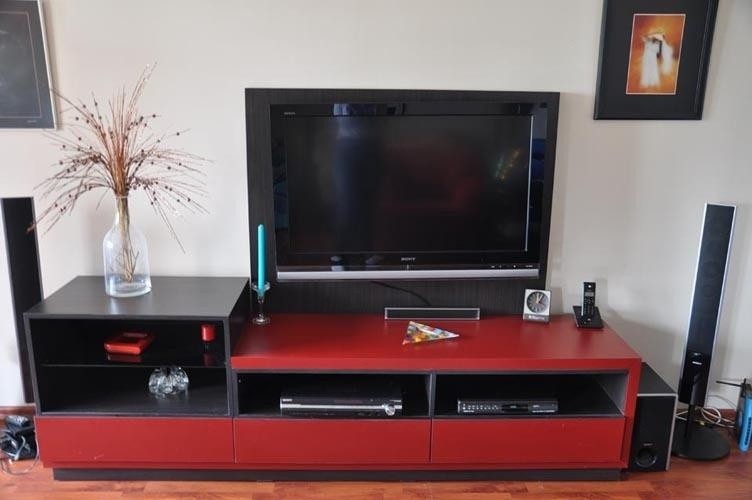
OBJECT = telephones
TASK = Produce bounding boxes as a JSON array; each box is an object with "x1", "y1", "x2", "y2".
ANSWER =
[{"x1": 573, "y1": 282, "x2": 603, "y2": 329}]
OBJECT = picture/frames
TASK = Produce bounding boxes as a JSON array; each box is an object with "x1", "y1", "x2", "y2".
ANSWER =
[
  {"x1": 0, "y1": 1, "x2": 60, "y2": 133},
  {"x1": 592, "y1": 0, "x2": 718, "y2": 119}
]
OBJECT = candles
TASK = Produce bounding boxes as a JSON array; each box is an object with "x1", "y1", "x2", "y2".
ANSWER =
[{"x1": 257, "y1": 223, "x2": 265, "y2": 288}]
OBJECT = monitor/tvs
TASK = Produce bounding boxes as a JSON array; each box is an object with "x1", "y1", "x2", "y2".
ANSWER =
[{"x1": 269, "y1": 101, "x2": 550, "y2": 284}]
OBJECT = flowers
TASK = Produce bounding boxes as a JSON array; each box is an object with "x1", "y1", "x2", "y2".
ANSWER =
[{"x1": 25, "y1": 60, "x2": 218, "y2": 284}]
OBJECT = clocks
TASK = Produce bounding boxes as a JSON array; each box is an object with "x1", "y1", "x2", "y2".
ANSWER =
[{"x1": 521, "y1": 288, "x2": 552, "y2": 323}]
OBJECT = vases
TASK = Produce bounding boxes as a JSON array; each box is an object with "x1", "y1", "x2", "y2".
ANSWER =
[{"x1": 102, "y1": 195, "x2": 154, "y2": 299}]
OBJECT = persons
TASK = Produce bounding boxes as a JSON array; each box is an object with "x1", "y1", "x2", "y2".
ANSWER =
[{"x1": 638, "y1": 29, "x2": 676, "y2": 88}]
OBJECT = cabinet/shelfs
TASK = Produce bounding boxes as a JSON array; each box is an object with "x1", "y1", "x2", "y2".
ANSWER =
[
  {"x1": 233, "y1": 311, "x2": 641, "y2": 482},
  {"x1": 24, "y1": 275, "x2": 249, "y2": 481}
]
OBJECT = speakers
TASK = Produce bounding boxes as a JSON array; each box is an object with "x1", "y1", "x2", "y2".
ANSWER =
[
  {"x1": 628, "y1": 362, "x2": 678, "y2": 473},
  {"x1": 0, "y1": 197, "x2": 46, "y2": 405},
  {"x1": 677, "y1": 201, "x2": 736, "y2": 461}
]
